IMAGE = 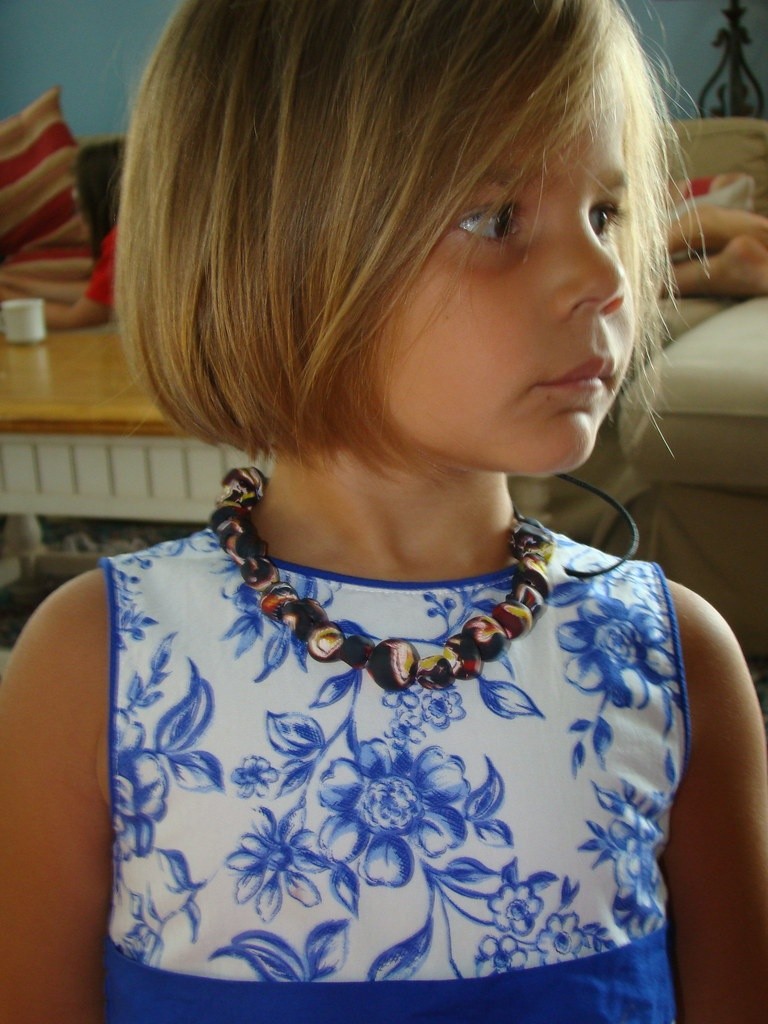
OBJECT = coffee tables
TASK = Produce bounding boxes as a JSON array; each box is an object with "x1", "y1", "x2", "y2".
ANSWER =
[{"x1": 0, "y1": 321, "x2": 276, "y2": 524}]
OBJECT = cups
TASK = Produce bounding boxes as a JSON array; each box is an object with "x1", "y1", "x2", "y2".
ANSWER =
[{"x1": 0, "y1": 296, "x2": 49, "y2": 344}]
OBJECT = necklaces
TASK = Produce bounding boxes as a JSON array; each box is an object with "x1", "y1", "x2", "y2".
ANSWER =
[{"x1": 211, "y1": 466, "x2": 554, "y2": 688}]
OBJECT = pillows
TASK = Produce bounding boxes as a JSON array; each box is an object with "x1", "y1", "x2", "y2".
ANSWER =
[{"x1": 0, "y1": 85, "x2": 94, "y2": 304}]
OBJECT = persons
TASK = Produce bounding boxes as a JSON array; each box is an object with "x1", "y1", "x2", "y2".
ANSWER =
[
  {"x1": 0, "y1": 0, "x2": 768, "y2": 1024},
  {"x1": 0, "y1": 138, "x2": 768, "y2": 327}
]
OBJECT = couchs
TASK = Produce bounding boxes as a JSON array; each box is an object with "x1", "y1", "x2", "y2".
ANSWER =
[{"x1": 73, "y1": 117, "x2": 767, "y2": 664}]
